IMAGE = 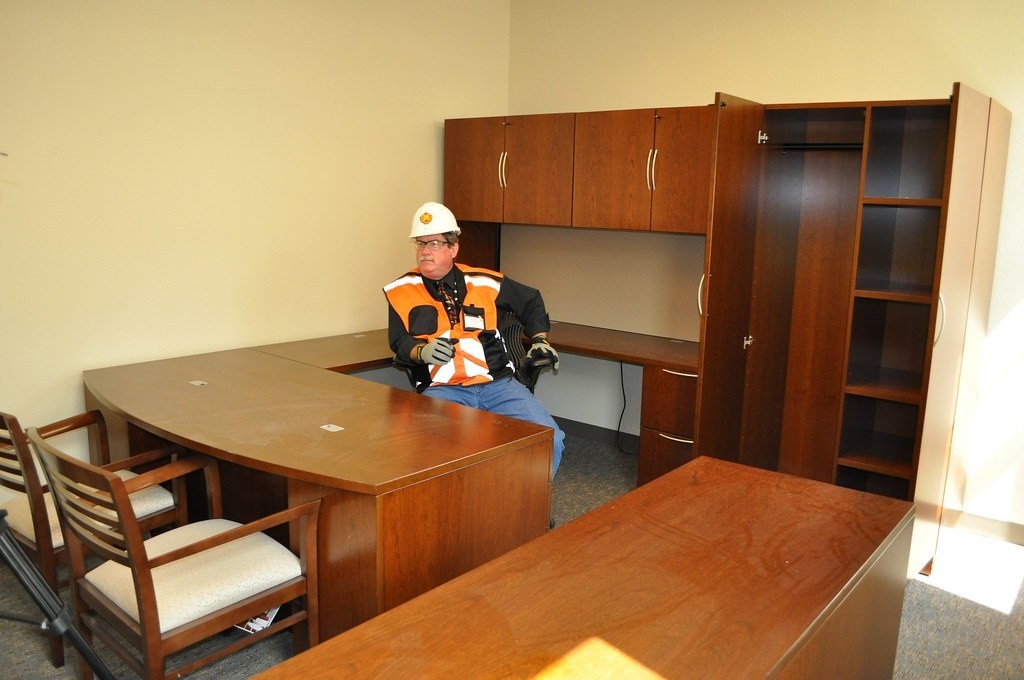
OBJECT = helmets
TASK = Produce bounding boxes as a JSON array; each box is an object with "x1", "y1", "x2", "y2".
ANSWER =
[{"x1": 408, "y1": 202, "x2": 462, "y2": 238}]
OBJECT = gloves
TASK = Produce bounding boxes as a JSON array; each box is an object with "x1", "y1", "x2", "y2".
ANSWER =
[
  {"x1": 417, "y1": 338, "x2": 460, "y2": 366},
  {"x1": 525, "y1": 335, "x2": 560, "y2": 375}
]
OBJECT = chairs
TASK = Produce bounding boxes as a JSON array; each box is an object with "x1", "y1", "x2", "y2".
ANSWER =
[
  {"x1": 393, "y1": 310, "x2": 553, "y2": 395},
  {"x1": 27, "y1": 426, "x2": 322, "y2": 680},
  {"x1": 0, "y1": 412, "x2": 188, "y2": 670}
]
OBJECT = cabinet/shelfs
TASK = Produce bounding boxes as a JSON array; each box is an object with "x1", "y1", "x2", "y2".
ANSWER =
[
  {"x1": 572, "y1": 105, "x2": 716, "y2": 234},
  {"x1": 443, "y1": 111, "x2": 574, "y2": 228},
  {"x1": 691, "y1": 80, "x2": 1011, "y2": 588}
]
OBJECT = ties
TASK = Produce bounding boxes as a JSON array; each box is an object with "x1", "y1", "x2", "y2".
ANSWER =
[{"x1": 436, "y1": 280, "x2": 459, "y2": 327}]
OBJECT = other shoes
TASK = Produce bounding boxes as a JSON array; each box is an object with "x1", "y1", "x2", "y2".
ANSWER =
[{"x1": 550, "y1": 517, "x2": 556, "y2": 529}]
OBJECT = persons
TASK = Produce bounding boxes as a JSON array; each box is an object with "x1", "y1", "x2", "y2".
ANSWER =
[{"x1": 381, "y1": 202, "x2": 566, "y2": 529}]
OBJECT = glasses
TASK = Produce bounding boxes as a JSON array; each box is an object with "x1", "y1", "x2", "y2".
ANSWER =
[{"x1": 413, "y1": 240, "x2": 450, "y2": 251}]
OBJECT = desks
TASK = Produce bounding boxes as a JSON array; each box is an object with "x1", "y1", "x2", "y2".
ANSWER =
[
  {"x1": 253, "y1": 328, "x2": 396, "y2": 373},
  {"x1": 500, "y1": 314, "x2": 700, "y2": 488},
  {"x1": 82, "y1": 348, "x2": 554, "y2": 654},
  {"x1": 247, "y1": 455, "x2": 917, "y2": 680}
]
